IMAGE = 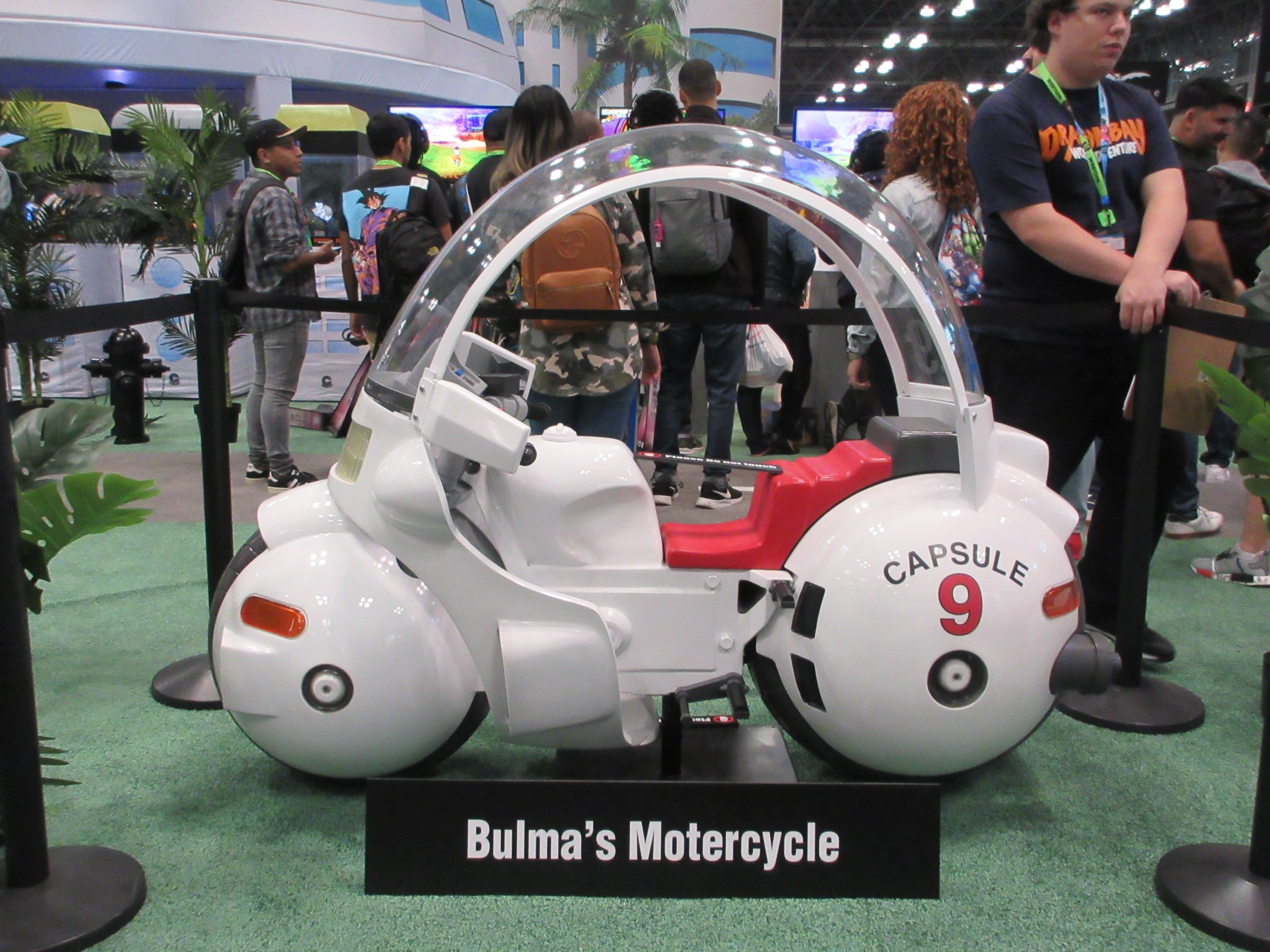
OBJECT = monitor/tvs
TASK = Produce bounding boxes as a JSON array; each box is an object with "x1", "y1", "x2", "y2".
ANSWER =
[
  {"x1": 793, "y1": 106, "x2": 895, "y2": 169},
  {"x1": 600, "y1": 105, "x2": 726, "y2": 137},
  {"x1": 385, "y1": 103, "x2": 515, "y2": 183}
]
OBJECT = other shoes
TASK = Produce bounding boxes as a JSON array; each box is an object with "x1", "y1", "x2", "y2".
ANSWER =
[{"x1": 776, "y1": 434, "x2": 801, "y2": 455}]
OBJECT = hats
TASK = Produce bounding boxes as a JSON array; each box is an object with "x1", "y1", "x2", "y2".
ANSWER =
[
  {"x1": 0, "y1": 126, "x2": 29, "y2": 147},
  {"x1": 243, "y1": 118, "x2": 309, "y2": 149}
]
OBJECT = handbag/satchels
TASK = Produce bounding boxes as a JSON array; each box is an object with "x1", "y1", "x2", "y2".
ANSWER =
[{"x1": 738, "y1": 323, "x2": 794, "y2": 389}]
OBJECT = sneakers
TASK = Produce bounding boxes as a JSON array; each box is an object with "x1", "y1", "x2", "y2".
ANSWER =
[
  {"x1": 1165, "y1": 505, "x2": 1225, "y2": 537},
  {"x1": 1204, "y1": 463, "x2": 1233, "y2": 485},
  {"x1": 244, "y1": 463, "x2": 269, "y2": 485},
  {"x1": 824, "y1": 399, "x2": 854, "y2": 452},
  {"x1": 1189, "y1": 545, "x2": 1270, "y2": 588},
  {"x1": 649, "y1": 471, "x2": 683, "y2": 505},
  {"x1": 696, "y1": 477, "x2": 743, "y2": 510},
  {"x1": 677, "y1": 432, "x2": 705, "y2": 454},
  {"x1": 266, "y1": 465, "x2": 319, "y2": 493},
  {"x1": 1139, "y1": 620, "x2": 1175, "y2": 663}
]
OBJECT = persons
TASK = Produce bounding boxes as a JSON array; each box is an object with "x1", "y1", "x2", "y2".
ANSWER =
[
  {"x1": 230, "y1": 119, "x2": 342, "y2": 492},
  {"x1": 459, "y1": 63, "x2": 815, "y2": 508},
  {"x1": 397, "y1": 113, "x2": 463, "y2": 236},
  {"x1": 337, "y1": 111, "x2": 453, "y2": 367},
  {"x1": 819, "y1": 81, "x2": 988, "y2": 447},
  {"x1": 1163, "y1": 79, "x2": 1269, "y2": 588},
  {"x1": 965, "y1": 0, "x2": 1205, "y2": 662}
]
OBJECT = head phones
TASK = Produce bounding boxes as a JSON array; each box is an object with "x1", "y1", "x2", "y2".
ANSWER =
[
  {"x1": 399, "y1": 112, "x2": 430, "y2": 153},
  {"x1": 850, "y1": 128, "x2": 892, "y2": 170},
  {"x1": 626, "y1": 88, "x2": 683, "y2": 131}
]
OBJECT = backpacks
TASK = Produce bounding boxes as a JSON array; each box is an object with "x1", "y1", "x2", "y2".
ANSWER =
[
  {"x1": 936, "y1": 200, "x2": 990, "y2": 308},
  {"x1": 520, "y1": 203, "x2": 625, "y2": 333},
  {"x1": 371, "y1": 172, "x2": 447, "y2": 360},
  {"x1": 649, "y1": 146, "x2": 736, "y2": 277},
  {"x1": 217, "y1": 177, "x2": 288, "y2": 316}
]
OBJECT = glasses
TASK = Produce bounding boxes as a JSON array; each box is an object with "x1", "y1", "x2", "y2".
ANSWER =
[{"x1": 261, "y1": 138, "x2": 301, "y2": 151}]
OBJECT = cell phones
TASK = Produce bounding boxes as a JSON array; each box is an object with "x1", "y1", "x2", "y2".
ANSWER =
[{"x1": 334, "y1": 246, "x2": 342, "y2": 251}]
